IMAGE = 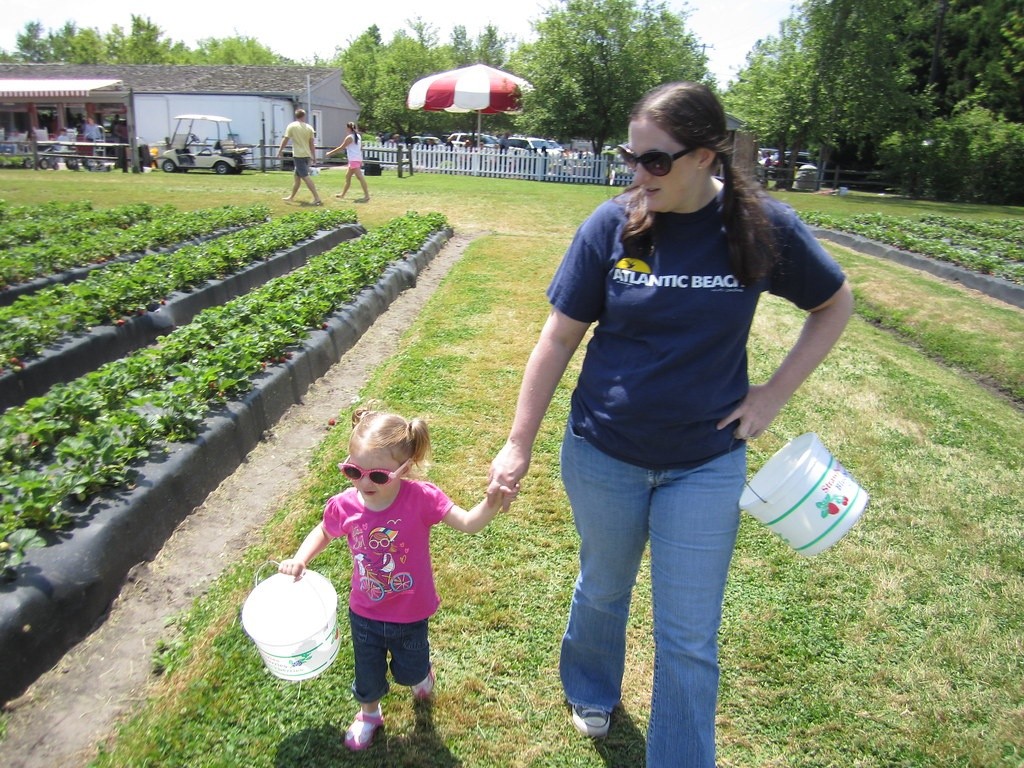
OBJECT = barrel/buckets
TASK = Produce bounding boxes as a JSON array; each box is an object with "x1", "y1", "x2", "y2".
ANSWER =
[
  {"x1": 361, "y1": 169, "x2": 365, "y2": 175},
  {"x1": 309, "y1": 164, "x2": 320, "y2": 176},
  {"x1": 243, "y1": 565, "x2": 340, "y2": 679},
  {"x1": 739, "y1": 430, "x2": 871, "y2": 558},
  {"x1": 143, "y1": 167, "x2": 152, "y2": 173}
]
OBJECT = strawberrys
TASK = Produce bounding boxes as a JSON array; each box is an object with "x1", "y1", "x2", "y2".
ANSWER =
[
  {"x1": 0, "y1": 256, "x2": 329, "y2": 554},
  {"x1": 329, "y1": 418, "x2": 335, "y2": 425}
]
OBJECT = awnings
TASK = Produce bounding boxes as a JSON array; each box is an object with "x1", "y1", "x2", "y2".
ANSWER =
[{"x1": 0, "y1": 80, "x2": 123, "y2": 97}]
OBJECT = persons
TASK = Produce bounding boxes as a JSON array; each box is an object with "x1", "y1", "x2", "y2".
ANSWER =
[
  {"x1": 5, "y1": 113, "x2": 128, "y2": 157},
  {"x1": 487, "y1": 78, "x2": 852, "y2": 768},
  {"x1": 277, "y1": 109, "x2": 321, "y2": 204},
  {"x1": 375, "y1": 131, "x2": 584, "y2": 171},
  {"x1": 277, "y1": 408, "x2": 521, "y2": 750},
  {"x1": 326, "y1": 122, "x2": 370, "y2": 201},
  {"x1": 760, "y1": 151, "x2": 771, "y2": 184}
]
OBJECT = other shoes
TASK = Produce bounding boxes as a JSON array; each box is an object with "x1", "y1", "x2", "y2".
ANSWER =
[
  {"x1": 410, "y1": 662, "x2": 435, "y2": 702},
  {"x1": 344, "y1": 711, "x2": 384, "y2": 752}
]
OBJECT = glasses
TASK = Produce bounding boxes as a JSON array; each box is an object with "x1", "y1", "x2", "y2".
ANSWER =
[
  {"x1": 338, "y1": 455, "x2": 410, "y2": 488},
  {"x1": 617, "y1": 144, "x2": 701, "y2": 177}
]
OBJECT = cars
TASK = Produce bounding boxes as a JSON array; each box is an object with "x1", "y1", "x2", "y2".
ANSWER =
[
  {"x1": 402, "y1": 134, "x2": 565, "y2": 169},
  {"x1": 758, "y1": 147, "x2": 816, "y2": 181}
]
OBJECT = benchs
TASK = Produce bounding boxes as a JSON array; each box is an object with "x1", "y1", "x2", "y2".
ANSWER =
[{"x1": 220, "y1": 141, "x2": 248, "y2": 154}]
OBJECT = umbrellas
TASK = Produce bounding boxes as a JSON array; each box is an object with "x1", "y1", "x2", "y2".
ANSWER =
[{"x1": 406, "y1": 64, "x2": 535, "y2": 147}]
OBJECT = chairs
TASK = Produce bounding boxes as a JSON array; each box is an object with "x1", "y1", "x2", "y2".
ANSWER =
[
  {"x1": 16, "y1": 131, "x2": 28, "y2": 142},
  {"x1": 64, "y1": 127, "x2": 78, "y2": 143},
  {"x1": 197, "y1": 141, "x2": 220, "y2": 155},
  {"x1": 32, "y1": 126, "x2": 49, "y2": 141}
]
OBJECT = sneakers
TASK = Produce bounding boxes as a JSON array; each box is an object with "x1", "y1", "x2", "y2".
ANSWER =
[{"x1": 572, "y1": 703, "x2": 610, "y2": 738}]
